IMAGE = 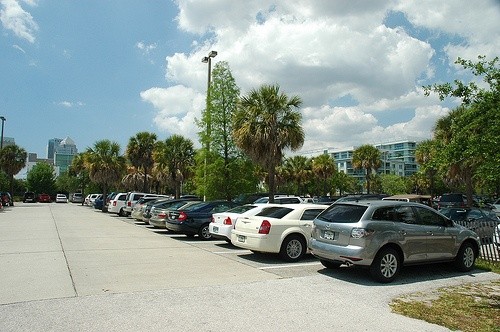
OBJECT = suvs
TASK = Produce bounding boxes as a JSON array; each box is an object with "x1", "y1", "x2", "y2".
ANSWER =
[{"x1": 23, "y1": 191, "x2": 37, "y2": 203}]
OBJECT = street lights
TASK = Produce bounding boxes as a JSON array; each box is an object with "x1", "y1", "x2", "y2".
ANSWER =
[
  {"x1": 0, "y1": 116, "x2": 7, "y2": 159},
  {"x1": 201, "y1": 50, "x2": 218, "y2": 202}
]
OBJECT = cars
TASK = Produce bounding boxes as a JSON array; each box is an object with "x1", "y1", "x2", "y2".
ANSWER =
[
  {"x1": 84, "y1": 191, "x2": 500, "y2": 241},
  {"x1": 308, "y1": 197, "x2": 482, "y2": 284},
  {"x1": 36, "y1": 193, "x2": 51, "y2": 203},
  {"x1": 208, "y1": 204, "x2": 263, "y2": 248},
  {"x1": 55, "y1": 193, "x2": 67, "y2": 203},
  {"x1": 231, "y1": 203, "x2": 332, "y2": 263},
  {"x1": 72, "y1": 192, "x2": 85, "y2": 203},
  {"x1": 0, "y1": 191, "x2": 13, "y2": 207}
]
